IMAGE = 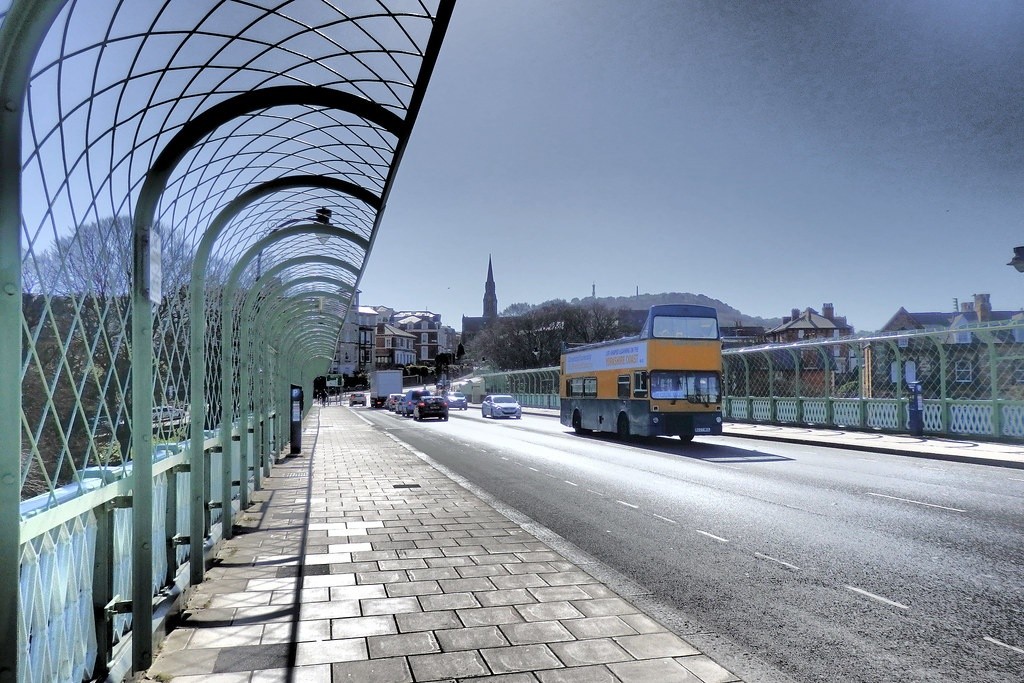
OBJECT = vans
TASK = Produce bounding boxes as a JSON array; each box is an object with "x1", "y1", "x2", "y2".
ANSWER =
[{"x1": 401, "y1": 388, "x2": 432, "y2": 417}]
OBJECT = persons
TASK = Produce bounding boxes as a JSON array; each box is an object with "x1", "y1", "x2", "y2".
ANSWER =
[{"x1": 321, "y1": 389, "x2": 328, "y2": 408}]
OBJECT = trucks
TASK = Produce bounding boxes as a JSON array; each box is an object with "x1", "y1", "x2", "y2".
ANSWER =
[{"x1": 369, "y1": 370, "x2": 404, "y2": 408}]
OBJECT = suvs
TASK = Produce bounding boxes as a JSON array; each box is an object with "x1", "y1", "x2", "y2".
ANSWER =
[{"x1": 349, "y1": 393, "x2": 367, "y2": 407}]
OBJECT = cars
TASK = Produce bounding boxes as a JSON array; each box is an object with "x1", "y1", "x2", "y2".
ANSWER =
[
  {"x1": 482, "y1": 394, "x2": 522, "y2": 419},
  {"x1": 384, "y1": 393, "x2": 406, "y2": 414},
  {"x1": 412, "y1": 395, "x2": 448, "y2": 421},
  {"x1": 444, "y1": 391, "x2": 468, "y2": 411}
]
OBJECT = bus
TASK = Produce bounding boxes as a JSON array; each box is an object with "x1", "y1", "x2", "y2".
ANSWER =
[{"x1": 557, "y1": 303, "x2": 726, "y2": 444}]
